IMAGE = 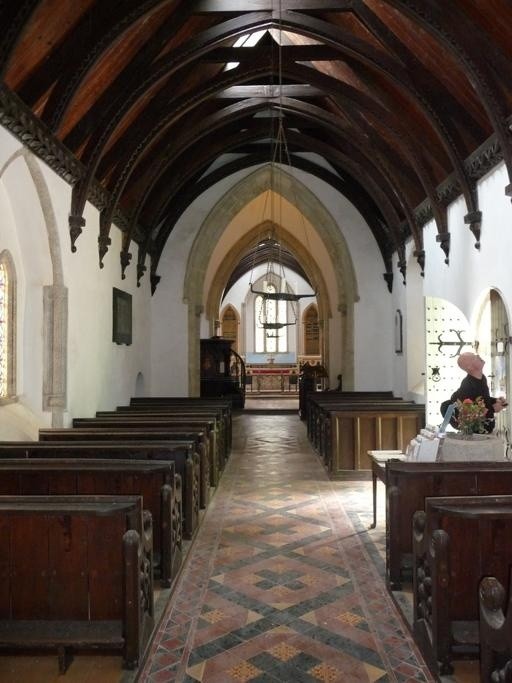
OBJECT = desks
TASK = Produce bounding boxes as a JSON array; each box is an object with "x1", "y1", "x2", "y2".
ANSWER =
[{"x1": 365, "y1": 448, "x2": 407, "y2": 530}]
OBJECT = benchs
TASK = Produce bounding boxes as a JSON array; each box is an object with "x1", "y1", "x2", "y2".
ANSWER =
[
  {"x1": 304, "y1": 388, "x2": 427, "y2": 482},
  {"x1": 383, "y1": 456, "x2": 512, "y2": 682}
]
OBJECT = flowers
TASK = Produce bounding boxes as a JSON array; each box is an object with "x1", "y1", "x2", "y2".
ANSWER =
[{"x1": 450, "y1": 396, "x2": 493, "y2": 435}]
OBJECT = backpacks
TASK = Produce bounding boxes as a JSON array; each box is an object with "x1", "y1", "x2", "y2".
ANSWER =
[{"x1": 440, "y1": 400, "x2": 462, "y2": 431}]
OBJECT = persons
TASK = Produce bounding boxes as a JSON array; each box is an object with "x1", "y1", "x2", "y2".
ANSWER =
[{"x1": 450, "y1": 352, "x2": 509, "y2": 433}]
